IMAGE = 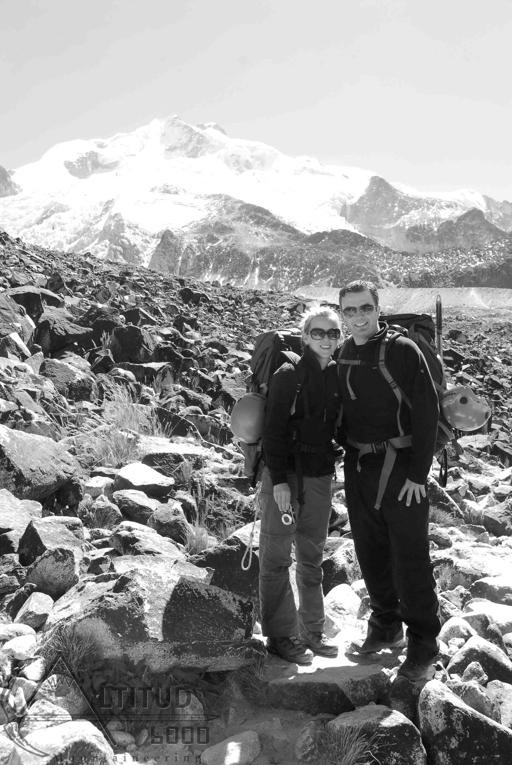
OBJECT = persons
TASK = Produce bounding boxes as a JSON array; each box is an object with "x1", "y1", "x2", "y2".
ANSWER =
[
  {"x1": 254, "y1": 307, "x2": 348, "y2": 669},
  {"x1": 332, "y1": 276, "x2": 440, "y2": 689}
]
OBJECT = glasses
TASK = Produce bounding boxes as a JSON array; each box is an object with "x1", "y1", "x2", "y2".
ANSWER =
[
  {"x1": 307, "y1": 327, "x2": 340, "y2": 340},
  {"x1": 340, "y1": 304, "x2": 374, "y2": 315}
]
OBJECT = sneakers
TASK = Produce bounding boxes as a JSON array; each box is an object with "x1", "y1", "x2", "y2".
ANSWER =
[{"x1": 266, "y1": 630, "x2": 338, "y2": 664}]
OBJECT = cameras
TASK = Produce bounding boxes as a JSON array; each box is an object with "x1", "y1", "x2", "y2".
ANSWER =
[{"x1": 279, "y1": 504, "x2": 296, "y2": 526}]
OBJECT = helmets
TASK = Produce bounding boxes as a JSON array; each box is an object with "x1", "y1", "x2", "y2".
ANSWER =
[
  {"x1": 440, "y1": 385, "x2": 492, "y2": 432},
  {"x1": 230, "y1": 392, "x2": 267, "y2": 445}
]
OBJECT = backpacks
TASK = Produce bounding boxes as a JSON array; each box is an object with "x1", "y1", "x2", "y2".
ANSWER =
[
  {"x1": 336, "y1": 313, "x2": 455, "y2": 453},
  {"x1": 244, "y1": 328, "x2": 343, "y2": 476}
]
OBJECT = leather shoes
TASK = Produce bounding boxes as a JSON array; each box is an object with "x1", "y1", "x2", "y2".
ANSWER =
[
  {"x1": 351, "y1": 635, "x2": 406, "y2": 654},
  {"x1": 398, "y1": 641, "x2": 440, "y2": 681}
]
